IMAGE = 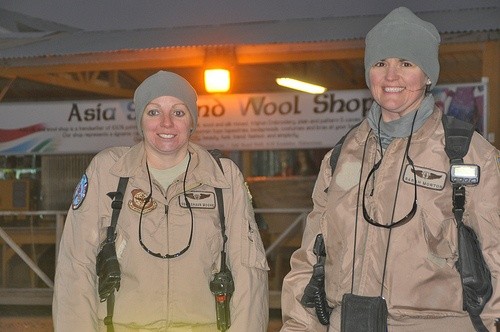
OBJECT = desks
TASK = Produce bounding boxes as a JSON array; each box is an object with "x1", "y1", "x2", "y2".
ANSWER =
[{"x1": 0, "y1": 225, "x2": 56, "y2": 284}]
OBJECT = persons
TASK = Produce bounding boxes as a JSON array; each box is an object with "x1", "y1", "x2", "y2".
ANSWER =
[
  {"x1": 52, "y1": 71, "x2": 271, "y2": 332},
  {"x1": 280, "y1": 7, "x2": 500, "y2": 332},
  {"x1": 273, "y1": 149, "x2": 319, "y2": 177}
]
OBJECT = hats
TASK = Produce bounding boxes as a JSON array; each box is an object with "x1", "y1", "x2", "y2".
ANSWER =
[
  {"x1": 364, "y1": 7, "x2": 440, "y2": 92},
  {"x1": 134, "y1": 71, "x2": 197, "y2": 136}
]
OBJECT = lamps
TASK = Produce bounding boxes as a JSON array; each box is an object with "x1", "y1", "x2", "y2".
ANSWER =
[{"x1": 200, "y1": 46, "x2": 238, "y2": 93}]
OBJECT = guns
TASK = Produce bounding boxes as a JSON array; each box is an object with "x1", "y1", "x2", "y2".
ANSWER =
[{"x1": 210, "y1": 271, "x2": 234, "y2": 331}]
